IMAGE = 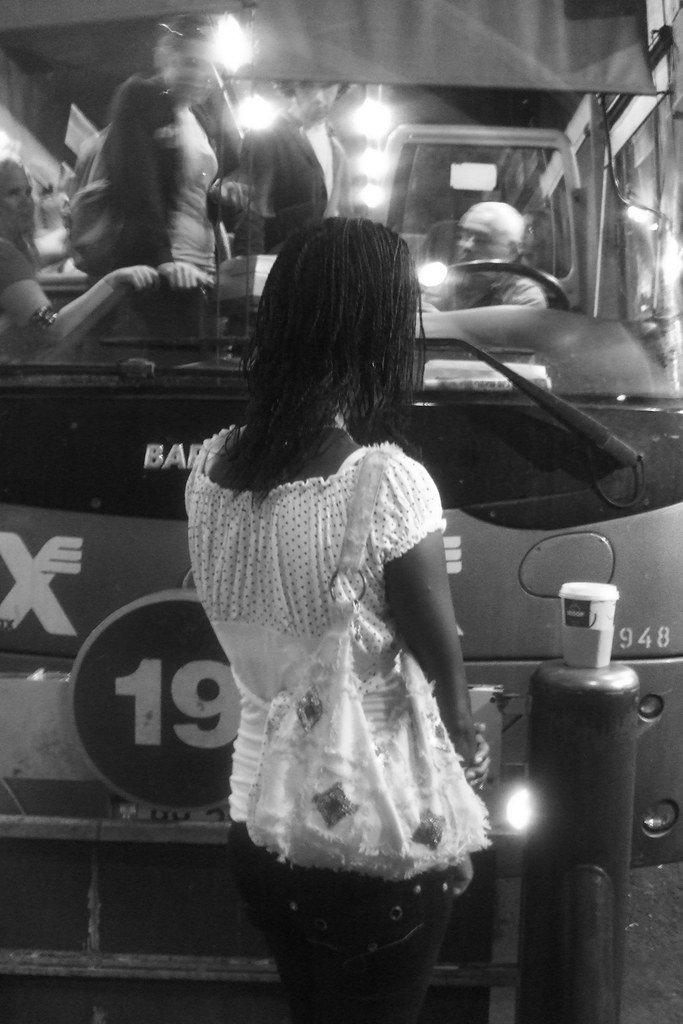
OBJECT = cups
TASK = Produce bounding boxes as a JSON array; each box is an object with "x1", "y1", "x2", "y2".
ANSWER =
[{"x1": 558, "y1": 582, "x2": 622, "y2": 669}]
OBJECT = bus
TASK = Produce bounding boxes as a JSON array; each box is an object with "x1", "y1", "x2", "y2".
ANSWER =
[{"x1": 2, "y1": 0, "x2": 682, "y2": 1024}]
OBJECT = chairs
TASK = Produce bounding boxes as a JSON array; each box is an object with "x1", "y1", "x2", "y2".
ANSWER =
[{"x1": 419, "y1": 219, "x2": 459, "y2": 263}]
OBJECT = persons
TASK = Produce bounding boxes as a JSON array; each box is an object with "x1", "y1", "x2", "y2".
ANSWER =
[
  {"x1": 0, "y1": 42, "x2": 353, "y2": 356},
  {"x1": 429, "y1": 200, "x2": 546, "y2": 309},
  {"x1": 181, "y1": 218, "x2": 492, "y2": 1024}
]
